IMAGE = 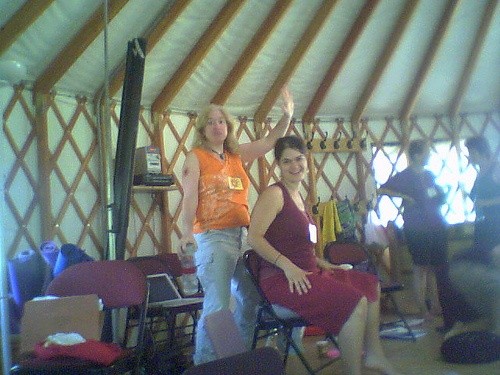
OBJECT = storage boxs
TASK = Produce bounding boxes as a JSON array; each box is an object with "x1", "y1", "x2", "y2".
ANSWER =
[{"x1": 133, "y1": 146, "x2": 161, "y2": 186}]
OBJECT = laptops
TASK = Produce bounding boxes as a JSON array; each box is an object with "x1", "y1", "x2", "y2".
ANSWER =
[{"x1": 145, "y1": 273, "x2": 204, "y2": 307}]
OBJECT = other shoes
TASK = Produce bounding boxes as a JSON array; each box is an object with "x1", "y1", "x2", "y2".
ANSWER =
[
  {"x1": 435, "y1": 324, "x2": 451, "y2": 333},
  {"x1": 432, "y1": 308, "x2": 443, "y2": 316},
  {"x1": 418, "y1": 312, "x2": 435, "y2": 322}
]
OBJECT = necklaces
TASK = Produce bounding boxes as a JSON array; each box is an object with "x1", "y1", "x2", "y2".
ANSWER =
[{"x1": 212, "y1": 149, "x2": 225, "y2": 160}]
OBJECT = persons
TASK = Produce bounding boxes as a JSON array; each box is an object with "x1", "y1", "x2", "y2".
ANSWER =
[
  {"x1": 248, "y1": 135, "x2": 400, "y2": 375},
  {"x1": 464, "y1": 135, "x2": 500, "y2": 265},
  {"x1": 377, "y1": 139, "x2": 447, "y2": 330},
  {"x1": 175, "y1": 86, "x2": 294, "y2": 365}
]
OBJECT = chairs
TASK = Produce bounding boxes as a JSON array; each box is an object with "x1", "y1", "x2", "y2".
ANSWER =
[{"x1": 9, "y1": 239, "x2": 418, "y2": 375}]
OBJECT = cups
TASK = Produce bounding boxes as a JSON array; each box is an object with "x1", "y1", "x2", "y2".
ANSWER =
[{"x1": 180, "y1": 243, "x2": 196, "y2": 274}]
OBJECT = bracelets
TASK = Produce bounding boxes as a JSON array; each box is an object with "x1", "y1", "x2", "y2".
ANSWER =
[{"x1": 274, "y1": 254, "x2": 283, "y2": 265}]
